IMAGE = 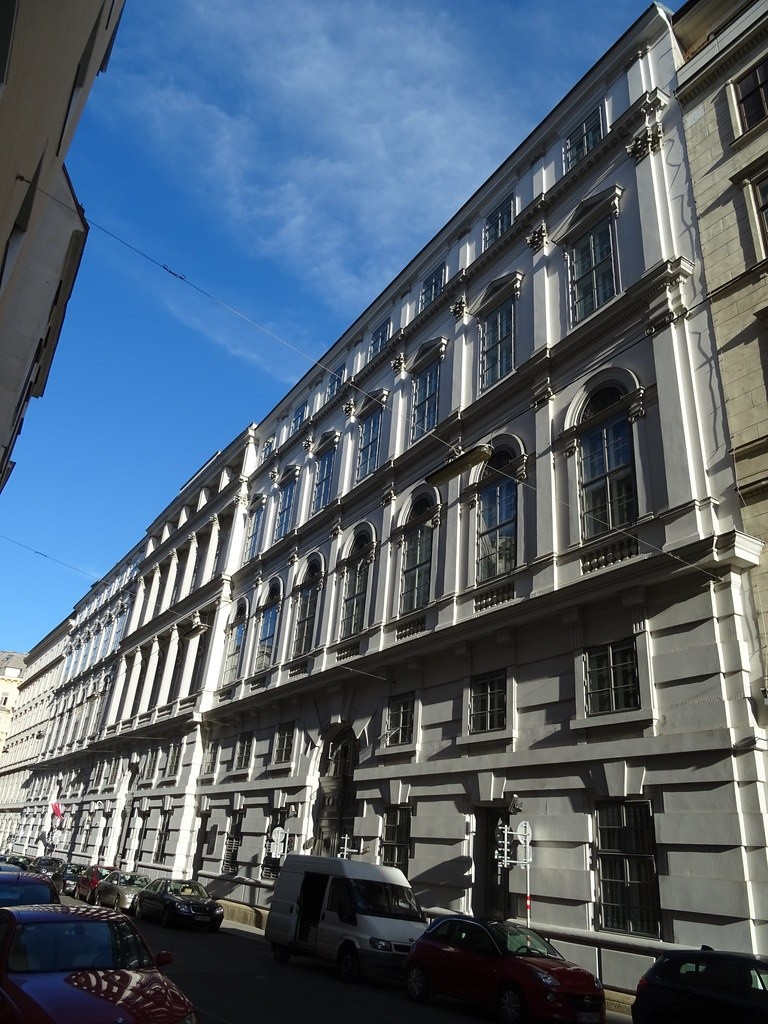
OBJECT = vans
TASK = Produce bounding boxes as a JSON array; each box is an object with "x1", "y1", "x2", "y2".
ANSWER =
[{"x1": 263, "y1": 853, "x2": 431, "y2": 979}]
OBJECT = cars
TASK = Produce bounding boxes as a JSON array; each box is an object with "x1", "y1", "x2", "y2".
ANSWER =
[
  {"x1": 75, "y1": 865, "x2": 124, "y2": 905},
  {"x1": 0, "y1": 864, "x2": 25, "y2": 902},
  {"x1": 399, "y1": 916, "x2": 606, "y2": 1024},
  {"x1": 0, "y1": 854, "x2": 36, "y2": 871},
  {"x1": 0, "y1": 870, "x2": 62, "y2": 957},
  {"x1": 138, "y1": 877, "x2": 225, "y2": 935},
  {"x1": 26, "y1": 856, "x2": 65, "y2": 880},
  {"x1": 0, "y1": 904, "x2": 200, "y2": 1024},
  {"x1": 50, "y1": 862, "x2": 90, "y2": 895},
  {"x1": 632, "y1": 945, "x2": 768, "y2": 1024},
  {"x1": 92, "y1": 871, "x2": 153, "y2": 913}
]
